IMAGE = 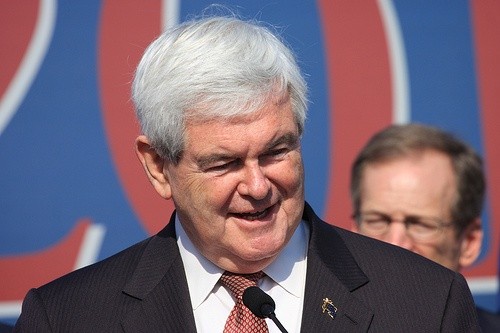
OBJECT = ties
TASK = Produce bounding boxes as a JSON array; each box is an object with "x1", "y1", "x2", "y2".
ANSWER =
[{"x1": 218, "y1": 269, "x2": 271, "y2": 333}]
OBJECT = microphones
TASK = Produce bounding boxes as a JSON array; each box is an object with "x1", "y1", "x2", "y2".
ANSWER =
[{"x1": 242, "y1": 286, "x2": 289, "y2": 333}]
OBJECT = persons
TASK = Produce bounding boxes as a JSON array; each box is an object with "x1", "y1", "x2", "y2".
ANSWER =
[
  {"x1": 13, "y1": 15, "x2": 483, "y2": 333},
  {"x1": 347, "y1": 122, "x2": 499, "y2": 333}
]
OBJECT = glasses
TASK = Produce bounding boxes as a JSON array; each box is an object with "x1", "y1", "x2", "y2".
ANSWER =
[{"x1": 350, "y1": 211, "x2": 463, "y2": 242}]
